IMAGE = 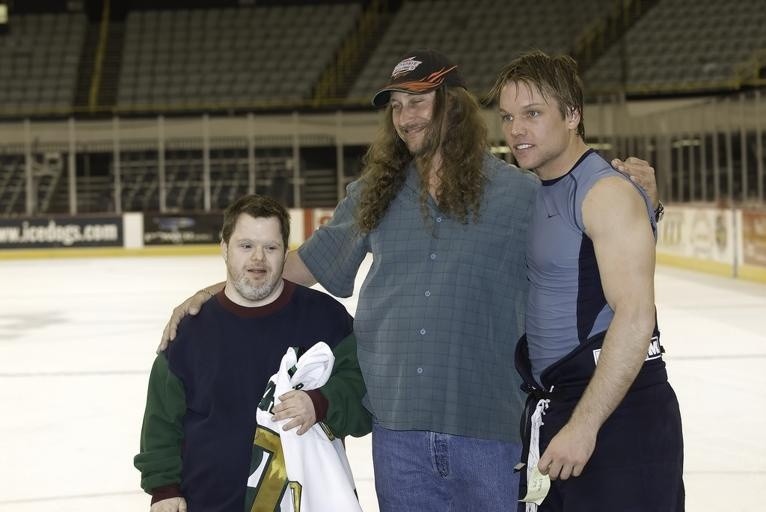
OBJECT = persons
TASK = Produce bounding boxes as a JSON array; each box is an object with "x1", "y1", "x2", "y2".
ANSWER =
[
  {"x1": 498, "y1": 52, "x2": 685, "y2": 512},
  {"x1": 134, "y1": 194, "x2": 373, "y2": 512},
  {"x1": 157, "y1": 48, "x2": 658, "y2": 512}
]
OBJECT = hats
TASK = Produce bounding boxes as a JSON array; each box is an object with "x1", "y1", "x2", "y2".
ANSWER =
[{"x1": 372, "y1": 50, "x2": 466, "y2": 107}]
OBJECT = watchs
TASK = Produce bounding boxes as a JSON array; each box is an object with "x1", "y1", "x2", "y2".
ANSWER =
[{"x1": 654, "y1": 201, "x2": 665, "y2": 223}]
